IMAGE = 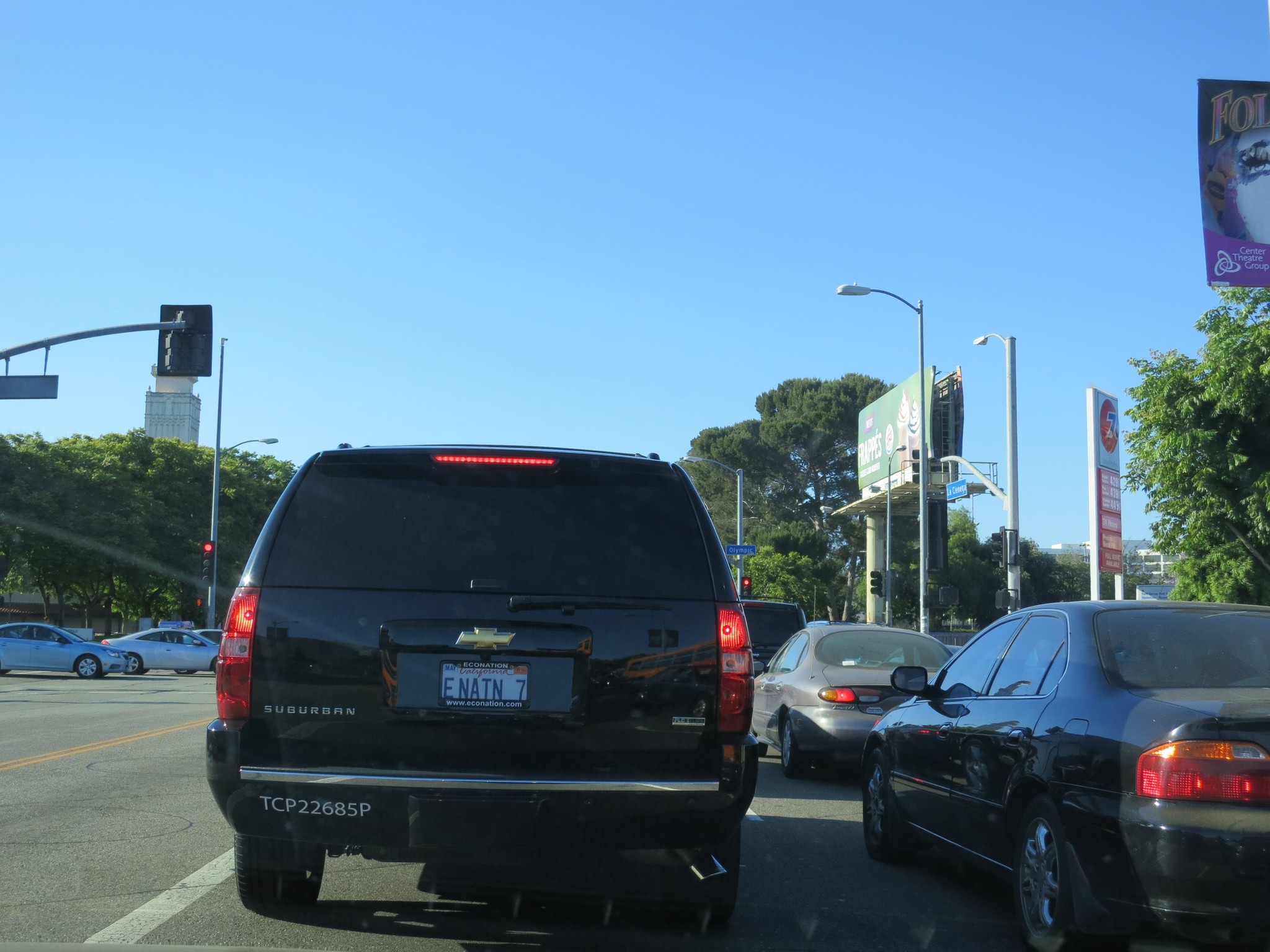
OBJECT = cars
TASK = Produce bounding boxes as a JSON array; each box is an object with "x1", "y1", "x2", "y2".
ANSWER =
[
  {"x1": 171, "y1": 629, "x2": 223, "y2": 675},
  {"x1": 882, "y1": 644, "x2": 962, "y2": 669},
  {"x1": 862, "y1": 599, "x2": 1270, "y2": 952},
  {"x1": 1, "y1": 622, "x2": 130, "y2": 680},
  {"x1": 101, "y1": 627, "x2": 221, "y2": 676},
  {"x1": 747, "y1": 624, "x2": 955, "y2": 779}
]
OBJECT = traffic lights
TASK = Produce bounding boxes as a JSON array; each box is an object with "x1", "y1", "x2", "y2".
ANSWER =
[
  {"x1": 196, "y1": 598, "x2": 205, "y2": 608},
  {"x1": 995, "y1": 590, "x2": 1011, "y2": 609},
  {"x1": 741, "y1": 576, "x2": 753, "y2": 601},
  {"x1": 912, "y1": 449, "x2": 931, "y2": 486},
  {"x1": 991, "y1": 526, "x2": 1006, "y2": 568},
  {"x1": 200, "y1": 540, "x2": 216, "y2": 586},
  {"x1": 870, "y1": 570, "x2": 883, "y2": 598}
]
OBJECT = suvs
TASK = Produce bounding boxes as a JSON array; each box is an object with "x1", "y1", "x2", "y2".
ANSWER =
[
  {"x1": 807, "y1": 620, "x2": 890, "y2": 628},
  {"x1": 200, "y1": 444, "x2": 763, "y2": 934}
]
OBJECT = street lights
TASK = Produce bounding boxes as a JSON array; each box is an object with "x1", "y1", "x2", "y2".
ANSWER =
[
  {"x1": 884, "y1": 446, "x2": 907, "y2": 627},
  {"x1": 973, "y1": 331, "x2": 1023, "y2": 616},
  {"x1": 204, "y1": 438, "x2": 280, "y2": 631},
  {"x1": 682, "y1": 456, "x2": 744, "y2": 601},
  {"x1": 836, "y1": 282, "x2": 931, "y2": 636}
]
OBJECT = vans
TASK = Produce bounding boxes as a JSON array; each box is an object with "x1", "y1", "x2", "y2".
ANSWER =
[{"x1": 738, "y1": 600, "x2": 808, "y2": 679}]
